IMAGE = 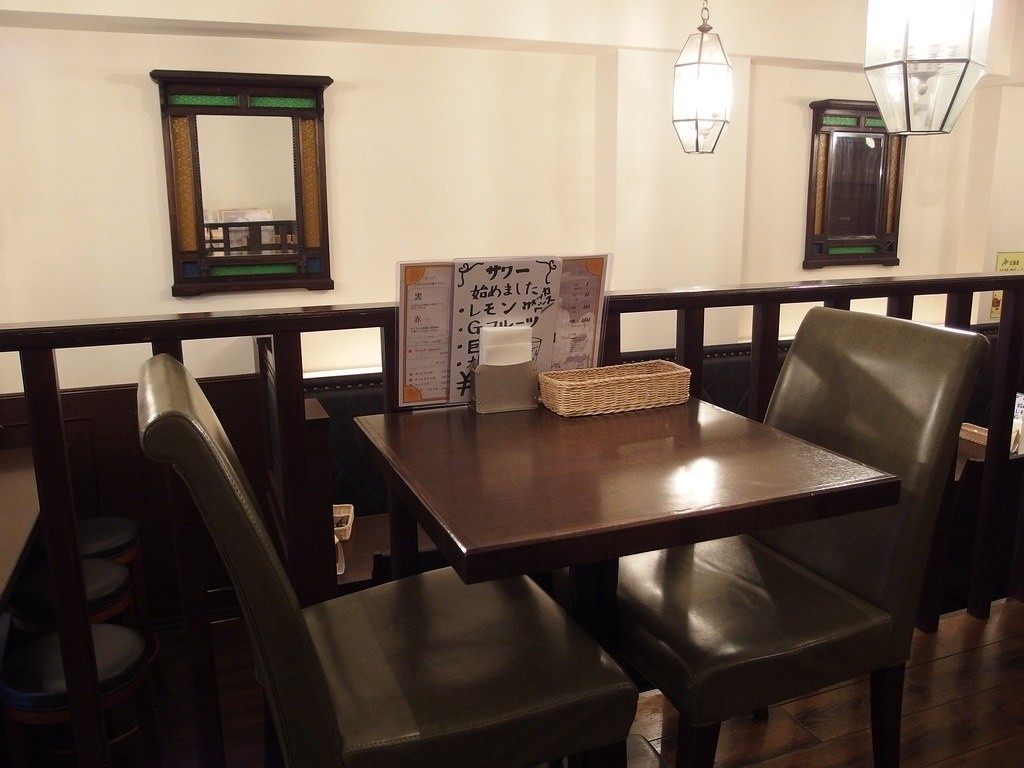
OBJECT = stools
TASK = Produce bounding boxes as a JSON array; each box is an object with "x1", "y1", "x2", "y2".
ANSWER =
[
  {"x1": 13, "y1": 558, "x2": 136, "y2": 636},
  {"x1": 0, "y1": 624, "x2": 161, "y2": 767},
  {"x1": 76, "y1": 517, "x2": 145, "y2": 591}
]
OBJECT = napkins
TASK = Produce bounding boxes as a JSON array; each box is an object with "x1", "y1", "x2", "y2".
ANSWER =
[{"x1": 479, "y1": 326, "x2": 534, "y2": 366}]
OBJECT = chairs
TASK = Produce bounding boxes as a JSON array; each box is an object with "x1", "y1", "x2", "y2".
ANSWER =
[
  {"x1": 617, "y1": 305, "x2": 989, "y2": 767},
  {"x1": 139, "y1": 353, "x2": 640, "y2": 767}
]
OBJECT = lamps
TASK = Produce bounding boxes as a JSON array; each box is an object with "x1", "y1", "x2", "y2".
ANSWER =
[
  {"x1": 865, "y1": 0, "x2": 993, "y2": 134},
  {"x1": 673, "y1": 0, "x2": 735, "y2": 154}
]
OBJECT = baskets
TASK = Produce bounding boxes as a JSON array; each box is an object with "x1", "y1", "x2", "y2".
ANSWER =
[{"x1": 538, "y1": 359, "x2": 692, "y2": 418}]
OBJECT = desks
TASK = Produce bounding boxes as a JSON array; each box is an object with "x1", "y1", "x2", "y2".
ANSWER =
[
  {"x1": 0, "y1": 446, "x2": 42, "y2": 609},
  {"x1": 353, "y1": 395, "x2": 902, "y2": 656}
]
